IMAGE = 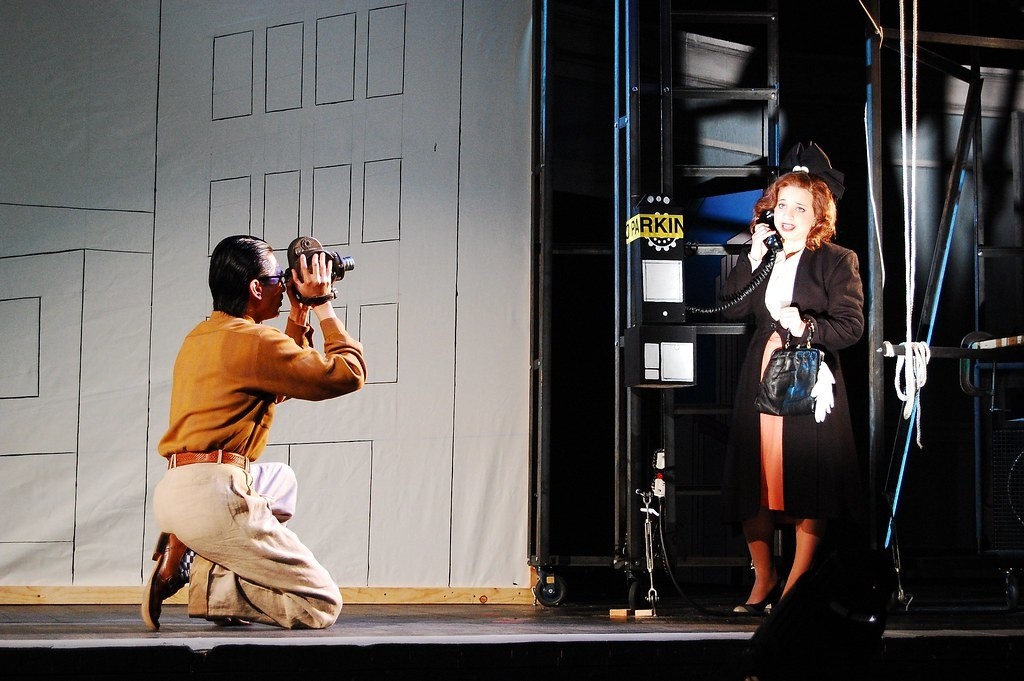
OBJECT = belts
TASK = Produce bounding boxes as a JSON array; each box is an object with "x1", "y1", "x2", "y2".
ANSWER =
[{"x1": 168, "y1": 451, "x2": 250, "y2": 472}]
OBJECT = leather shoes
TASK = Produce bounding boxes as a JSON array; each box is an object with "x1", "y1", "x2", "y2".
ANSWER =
[
  {"x1": 213, "y1": 615, "x2": 253, "y2": 627},
  {"x1": 141, "y1": 532, "x2": 188, "y2": 631}
]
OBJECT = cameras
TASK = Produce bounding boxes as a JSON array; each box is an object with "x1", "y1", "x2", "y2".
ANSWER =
[{"x1": 284, "y1": 236, "x2": 355, "y2": 286}]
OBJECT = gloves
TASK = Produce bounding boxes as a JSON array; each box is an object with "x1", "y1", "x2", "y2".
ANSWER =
[{"x1": 810, "y1": 362, "x2": 835, "y2": 423}]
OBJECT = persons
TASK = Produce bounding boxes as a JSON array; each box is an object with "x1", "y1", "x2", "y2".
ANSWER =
[
  {"x1": 140, "y1": 234, "x2": 367, "y2": 631},
  {"x1": 721, "y1": 171, "x2": 865, "y2": 617}
]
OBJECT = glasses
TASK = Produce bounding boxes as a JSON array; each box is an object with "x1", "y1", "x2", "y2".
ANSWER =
[{"x1": 254, "y1": 271, "x2": 291, "y2": 284}]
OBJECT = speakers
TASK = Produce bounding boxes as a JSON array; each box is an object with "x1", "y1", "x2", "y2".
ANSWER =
[{"x1": 973, "y1": 362, "x2": 1024, "y2": 555}]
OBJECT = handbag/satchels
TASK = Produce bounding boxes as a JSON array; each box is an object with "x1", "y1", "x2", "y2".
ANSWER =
[{"x1": 754, "y1": 318, "x2": 824, "y2": 417}]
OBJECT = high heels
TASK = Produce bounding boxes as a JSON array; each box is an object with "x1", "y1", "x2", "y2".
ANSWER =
[{"x1": 732, "y1": 576, "x2": 781, "y2": 615}]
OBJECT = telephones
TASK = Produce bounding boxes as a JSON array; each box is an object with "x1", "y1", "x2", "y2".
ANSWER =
[{"x1": 752, "y1": 209, "x2": 783, "y2": 251}]
